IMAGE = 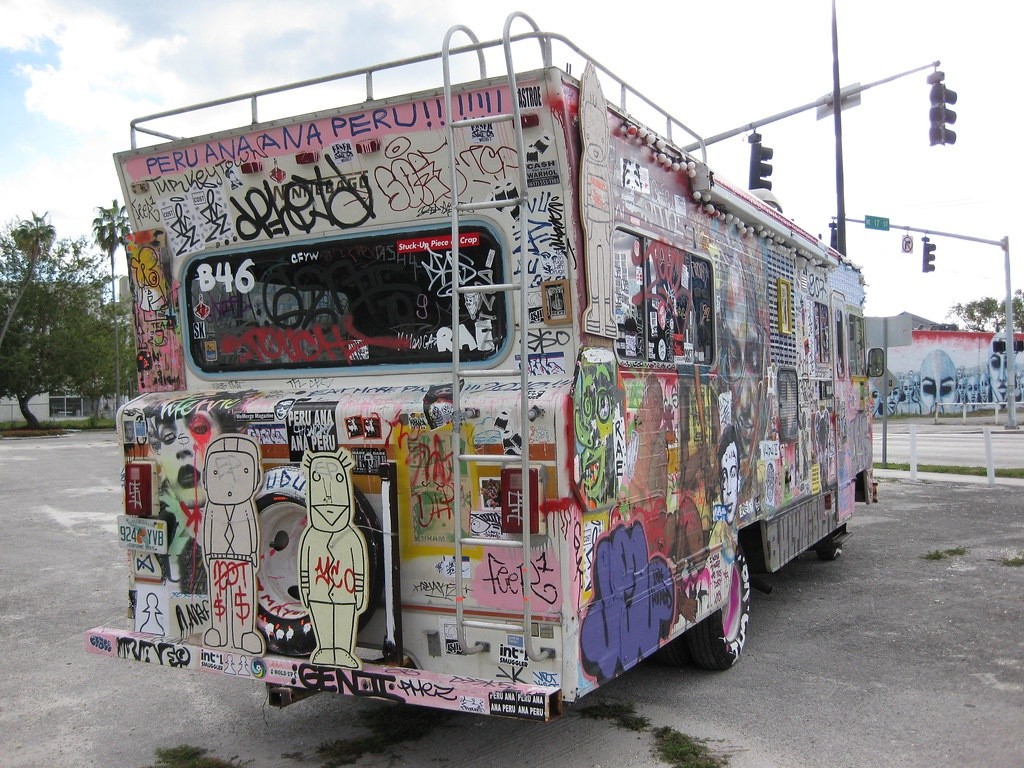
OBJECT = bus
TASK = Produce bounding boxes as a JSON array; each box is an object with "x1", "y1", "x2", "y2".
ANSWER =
[{"x1": 87, "y1": 11, "x2": 888, "y2": 722}]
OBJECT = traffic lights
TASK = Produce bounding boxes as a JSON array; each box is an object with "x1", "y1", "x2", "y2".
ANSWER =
[
  {"x1": 993, "y1": 341, "x2": 1006, "y2": 353},
  {"x1": 749, "y1": 143, "x2": 774, "y2": 191},
  {"x1": 922, "y1": 243, "x2": 936, "y2": 272},
  {"x1": 928, "y1": 82, "x2": 957, "y2": 146}
]
